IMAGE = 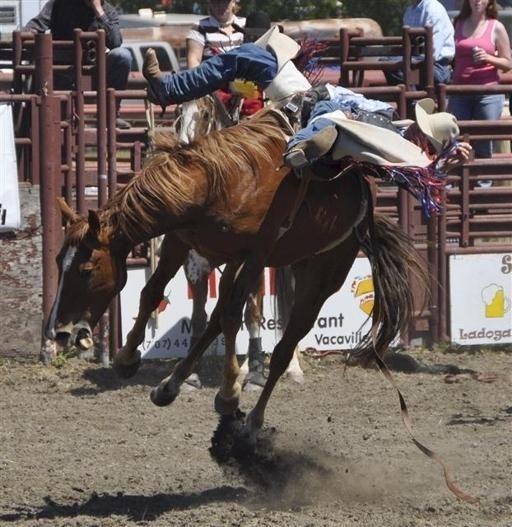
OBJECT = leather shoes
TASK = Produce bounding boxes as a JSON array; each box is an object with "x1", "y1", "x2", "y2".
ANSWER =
[
  {"x1": 286, "y1": 126, "x2": 338, "y2": 169},
  {"x1": 142, "y1": 49, "x2": 162, "y2": 106},
  {"x1": 116, "y1": 116, "x2": 129, "y2": 129}
]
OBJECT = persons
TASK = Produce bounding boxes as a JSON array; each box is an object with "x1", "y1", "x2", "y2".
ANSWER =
[
  {"x1": 379, "y1": 0, "x2": 455, "y2": 120},
  {"x1": 23, "y1": 0, "x2": 132, "y2": 130},
  {"x1": 186, "y1": 1, "x2": 265, "y2": 122},
  {"x1": 140, "y1": 25, "x2": 471, "y2": 170},
  {"x1": 442, "y1": 1, "x2": 511, "y2": 215}
]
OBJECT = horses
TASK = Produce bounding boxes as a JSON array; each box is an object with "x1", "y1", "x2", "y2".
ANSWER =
[
  {"x1": 45, "y1": 107, "x2": 444, "y2": 464},
  {"x1": 168, "y1": 92, "x2": 305, "y2": 390}
]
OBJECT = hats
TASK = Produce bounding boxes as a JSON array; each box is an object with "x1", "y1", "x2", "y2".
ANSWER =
[{"x1": 415, "y1": 98, "x2": 460, "y2": 151}]
